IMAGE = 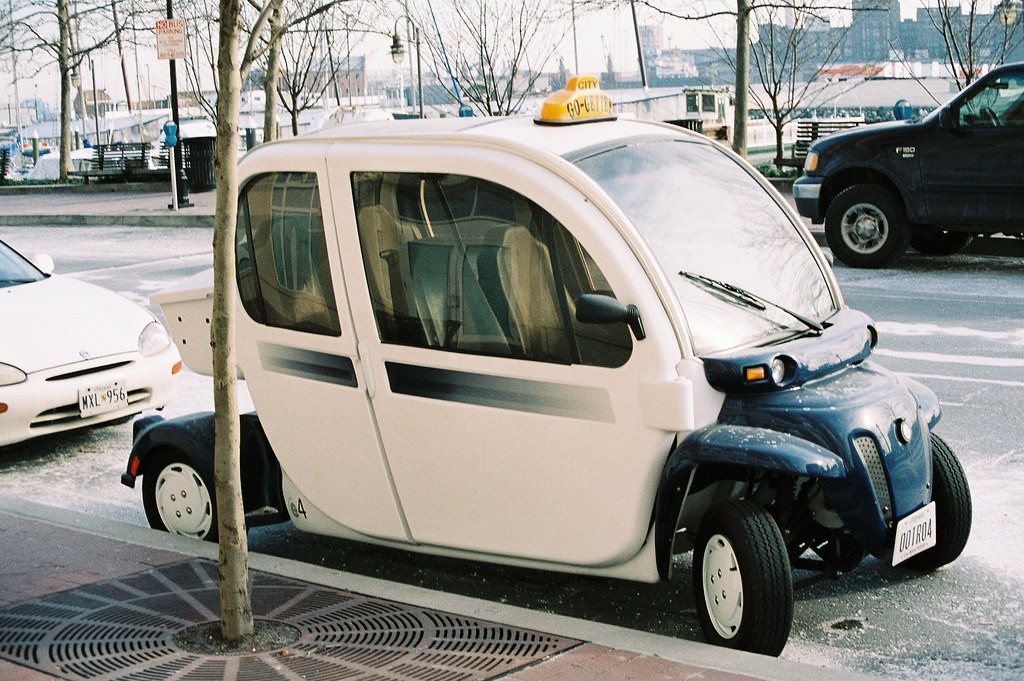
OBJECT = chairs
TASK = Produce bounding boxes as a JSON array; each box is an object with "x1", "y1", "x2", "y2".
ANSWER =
[
  {"x1": 475, "y1": 225, "x2": 579, "y2": 364},
  {"x1": 356, "y1": 203, "x2": 423, "y2": 345}
]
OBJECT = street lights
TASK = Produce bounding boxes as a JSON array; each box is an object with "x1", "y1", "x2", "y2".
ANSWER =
[{"x1": 392, "y1": 13, "x2": 425, "y2": 119}]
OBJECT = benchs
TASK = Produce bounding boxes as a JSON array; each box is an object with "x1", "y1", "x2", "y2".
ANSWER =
[
  {"x1": 67, "y1": 142, "x2": 151, "y2": 186},
  {"x1": 774, "y1": 120, "x2": 876, "y2": 178}
]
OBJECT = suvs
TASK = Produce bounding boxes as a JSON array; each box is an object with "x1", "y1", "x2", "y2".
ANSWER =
[{"x1": 791, "y1": 62, "x2": 1024, "y2": 268}]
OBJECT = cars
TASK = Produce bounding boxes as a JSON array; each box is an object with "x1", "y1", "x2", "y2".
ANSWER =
[
  {"x1": 0, "y1": 239, "x2": 178, "y2": 456},
  {"x1": 122, "y1": 77, "x2": 972, "y2": 659}
]
